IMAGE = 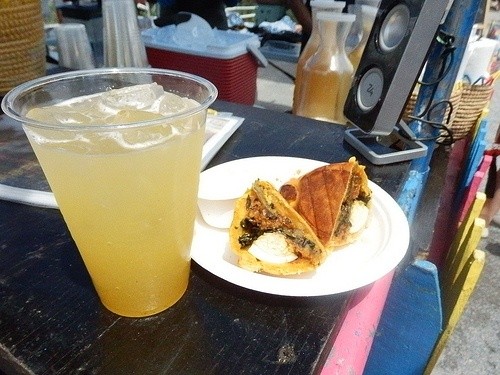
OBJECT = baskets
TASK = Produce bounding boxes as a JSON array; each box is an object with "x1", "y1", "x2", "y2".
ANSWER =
[{"x1": 404, "y1": 75, "x2": 495, "y2": 139}]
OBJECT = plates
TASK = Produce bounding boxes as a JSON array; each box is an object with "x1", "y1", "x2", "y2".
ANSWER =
[{"x1": 192, "y1": 156, "x2": 411, "y2": 298}]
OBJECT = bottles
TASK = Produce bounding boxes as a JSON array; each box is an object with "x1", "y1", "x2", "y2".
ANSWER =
[{"x1": 292, "y1": 0, "x2": 382, "y2": 124}]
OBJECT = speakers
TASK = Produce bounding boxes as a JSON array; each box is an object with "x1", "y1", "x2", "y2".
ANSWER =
[{"x1": 343, "y1": 0, "x2": 450, "y2": 135}]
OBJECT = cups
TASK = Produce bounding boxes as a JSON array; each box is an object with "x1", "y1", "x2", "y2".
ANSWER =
[
  {"x1": 55, "y1": 23, "x2": 95, "y2": 71},
  {"x1": 102, "y1": 0, "x2": 150, "y2": 67},
  {"x1": 1, "y1": 68, "x2": 219, "y2": 318}
]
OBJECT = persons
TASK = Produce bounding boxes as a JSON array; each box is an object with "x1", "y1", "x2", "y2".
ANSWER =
[
  {"x1": 480, "y1": 128, "x2": 500, "y2": 237},
  {"x1": 132, "y1": 0, "x2": 356, "y2": 60}
]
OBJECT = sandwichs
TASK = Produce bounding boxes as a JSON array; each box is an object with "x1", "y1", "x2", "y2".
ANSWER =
[
  {"x1": 230, "y1": 178, "x2": 326, "y2": 276},
  {"x1": 277, "y1": 157, "x2": 371, "y2": 245}
]
